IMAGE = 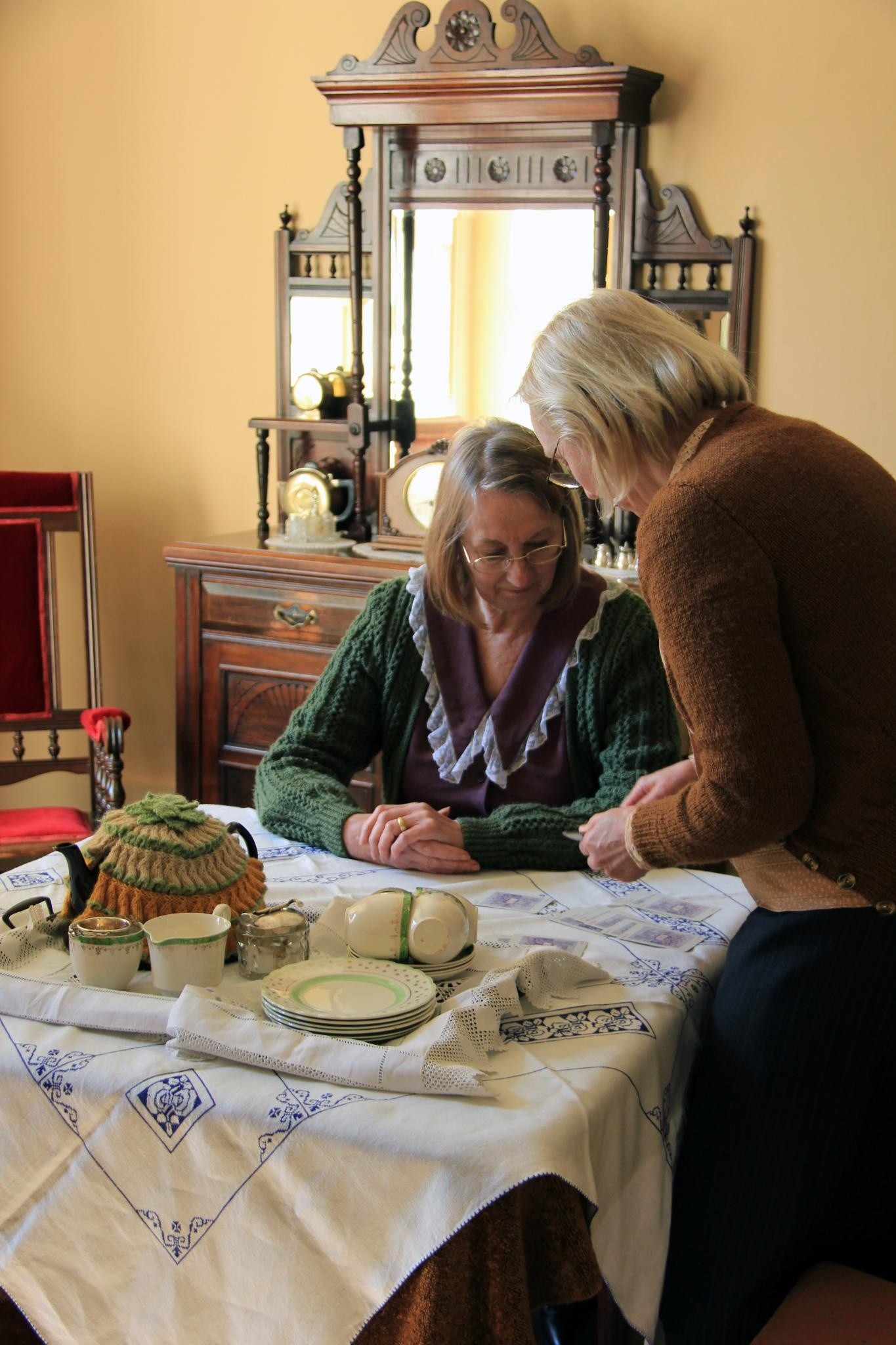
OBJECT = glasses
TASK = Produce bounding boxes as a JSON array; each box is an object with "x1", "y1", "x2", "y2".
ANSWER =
[
  {"x1": 545, "y1": 438, "x2": 580, "y2": 489},
  {"x1": 460, "y1": 521, "x2": 568, "y2": 575}
]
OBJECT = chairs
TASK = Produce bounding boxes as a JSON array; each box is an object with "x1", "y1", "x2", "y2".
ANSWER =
[{"x1": 0, "y1": 470, "x2": 130, "y2": 873}]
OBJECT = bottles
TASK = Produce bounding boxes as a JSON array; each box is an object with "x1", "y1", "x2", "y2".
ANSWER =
[
  {"x1": 237, "y1": 906, "x2": 310, "y2": 978},
  {"x1": 591, "y1": 541, "x2": 639, "y2": 571}
]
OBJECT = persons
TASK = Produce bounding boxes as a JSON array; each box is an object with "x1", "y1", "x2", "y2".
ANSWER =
[
  {"x1": 516, "y1": 288, "x2": 895, "y2": 1344},
  {"x1": 254, "y1": 421, "x2": 682, "y2": 876}
]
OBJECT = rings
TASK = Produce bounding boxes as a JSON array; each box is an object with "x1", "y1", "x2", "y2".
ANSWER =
[{"x1": 398, "y1": 817, "x2": 406, "y2": 831}]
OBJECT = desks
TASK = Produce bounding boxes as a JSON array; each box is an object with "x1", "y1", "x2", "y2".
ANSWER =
[{"x1": 0, "y1": 802, "x2": 762, "y2": 1345}]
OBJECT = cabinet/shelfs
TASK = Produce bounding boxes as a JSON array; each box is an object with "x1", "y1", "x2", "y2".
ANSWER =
[{"x1": 159, "y1": 523, "x2": 408, "y2": 806}]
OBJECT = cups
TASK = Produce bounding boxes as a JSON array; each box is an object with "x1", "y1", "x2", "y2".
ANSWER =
[
  {"x1": 142, "y1": 904, "x2": 231, "y2": 1000},
  {"x1": 68, "y1": 917, "x2": 145, "y2": 991},
  {"x1": 343, "y1": 883, "x2": 478, "y2": 966}
]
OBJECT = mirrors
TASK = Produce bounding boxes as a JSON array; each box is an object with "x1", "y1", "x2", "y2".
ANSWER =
[{"x1": 254, "y1": 122, "x2": 638, "y2": 586}]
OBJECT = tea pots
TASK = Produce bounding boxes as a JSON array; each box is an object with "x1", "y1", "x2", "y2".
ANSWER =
[{"x1": 51, "y1": 821, "x2": 260, "y2": 971}]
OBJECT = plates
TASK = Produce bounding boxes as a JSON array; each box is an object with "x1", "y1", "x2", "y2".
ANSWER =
[
  {"x1": 346, "y1": 944, "x2": 476, "y2": 981},
  {"x1": 260, "y1": 955, "x2": 438, "y2": 1042}
]
